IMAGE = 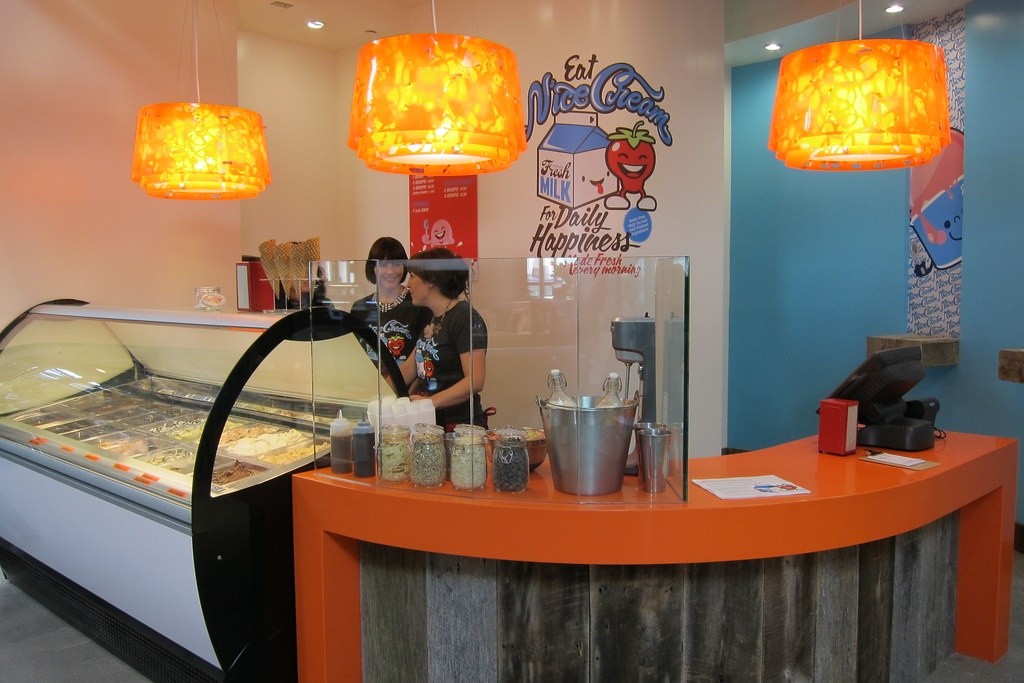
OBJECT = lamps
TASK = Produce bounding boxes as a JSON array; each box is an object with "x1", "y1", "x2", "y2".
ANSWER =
[
  {"x1": 767, "y1": 0, "x2": 951, "y2": 171},
  {"x1": 131, "y1": 0, "x2": 271, "y2": 200},
  {"x1": 346, "y1": 0, "x2": 527, "y2": 175}
]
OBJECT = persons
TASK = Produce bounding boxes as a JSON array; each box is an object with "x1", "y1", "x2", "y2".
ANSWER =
[
  {"x1": 384, "y1": 248, "x2": 496, "y2": 433},
  {"x1": 351, "y1": 238, "x2": 434, "y2": 396}
]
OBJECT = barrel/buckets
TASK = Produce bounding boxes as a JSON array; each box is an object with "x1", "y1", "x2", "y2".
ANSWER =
[{"x1": 534, "y1": 393, "x2": 639, "y2": 497}]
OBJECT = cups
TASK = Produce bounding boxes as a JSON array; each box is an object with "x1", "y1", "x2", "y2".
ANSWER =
[
  {"x1": 637, "y1": 429, "x2": 672, "y2": 493},
  {"x1": 633, "y1": 422, "x2": 667, "y2": 483}
]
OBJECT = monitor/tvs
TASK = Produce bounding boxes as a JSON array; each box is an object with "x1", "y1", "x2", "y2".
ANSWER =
[{"x1": 816, "y1": 345, "x2": 926, "y2": 424}]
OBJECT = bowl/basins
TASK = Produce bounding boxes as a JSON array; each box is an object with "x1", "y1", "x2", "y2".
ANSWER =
[{"x1": 486, "y1": 427, "x2": 547, "y2": 472}]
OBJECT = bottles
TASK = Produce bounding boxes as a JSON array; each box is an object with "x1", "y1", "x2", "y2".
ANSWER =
[
  {"x1": 441, "y1": 423, "x2": 489, "y2": 493},
  {"x1": 351, "y1": 412, "x2": 375, "y2": 478},
  {"x1": 329, "y1": 409, "x2": 354, "y2": 474},
  {"x1": 406, "y1": 421, "x2": 449, "y2": 490},
  {"x1": 192, "y1": 287, "x2": 223, "y2": 313},
  {"x1": 491, "y1": 427, "x2": 532, "y2": 494},
  {"x1": 375, "y1": 424, "x2": 412, "y2": 481}
]
